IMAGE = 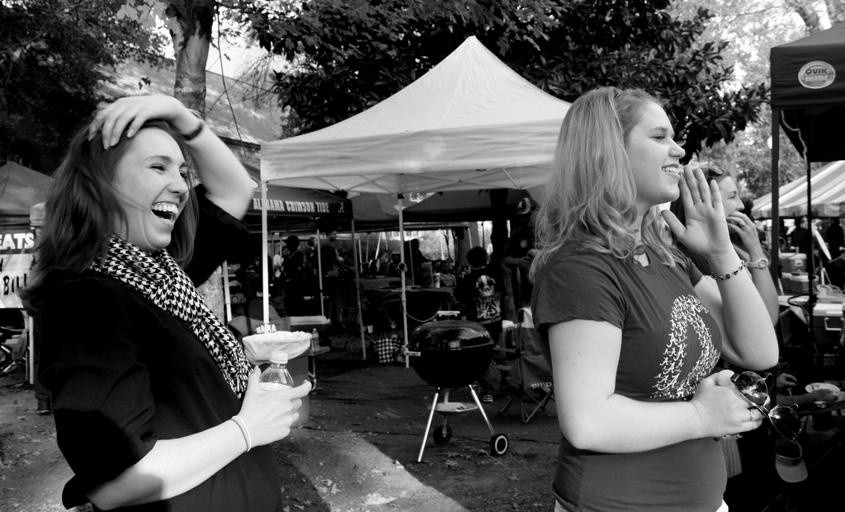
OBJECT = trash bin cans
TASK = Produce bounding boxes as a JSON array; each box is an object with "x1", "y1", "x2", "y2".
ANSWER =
[{"x1": 244, "y1": 333, "x2": 309, "y2": 427}]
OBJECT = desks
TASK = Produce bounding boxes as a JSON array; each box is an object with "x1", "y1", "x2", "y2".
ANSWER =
[
  {"x1": 303, "y1": 346, "x2": 332, "y2": 392},
  {"x1": 354, "y1": 285, "x2": 457, "y2": 367}
]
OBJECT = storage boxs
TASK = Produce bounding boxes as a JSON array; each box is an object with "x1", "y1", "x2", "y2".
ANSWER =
[
  {"x1": 774, "y1": 295, "x2": 813, "y2": 341},
  {"x1": 812, "y1": 301, "x2": 844, "y2": 351},
  {"x1": 779, "y1": 251, "x2": 821, "y2": 295},
  {"x1": 289, "y1": 315, "x2": 329, "y2": 342}
]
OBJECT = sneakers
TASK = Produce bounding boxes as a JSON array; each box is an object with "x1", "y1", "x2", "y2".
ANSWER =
[{"x1": 478, "y1": 389, "x2": 493, "y2": 402}]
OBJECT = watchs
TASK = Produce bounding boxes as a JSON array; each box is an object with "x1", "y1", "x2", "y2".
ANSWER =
[{"x1": 748, "y1": 258, "x2": 769, "y2": 269}]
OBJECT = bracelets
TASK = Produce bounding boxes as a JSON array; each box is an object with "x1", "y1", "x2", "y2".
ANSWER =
[
  {"x1": 179, "y1": 109, "x2": 204, "y2": 139},
  {"x1": 231, "y1": 416, "x2": 251, "y2": 452},
  {"x1": 711, "y1": 260, "x2": 745, "y2": 280}
]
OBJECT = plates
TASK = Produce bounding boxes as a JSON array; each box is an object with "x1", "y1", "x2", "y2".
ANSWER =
[{"x1": 805, "y1": 382, "x2": 842, "y2": 397}]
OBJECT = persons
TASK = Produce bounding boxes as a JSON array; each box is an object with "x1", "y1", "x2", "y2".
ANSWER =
[
  {"x1": 18, "y1": 92, "x2": 313, "y2": 512},
  {"x1": 671, "y1": 163, "x2": 779, "y2": 480},
  {"x1": 739, "y1": 197, "x2": 765, "y2": 241},
  {"x1": 778, "y1": 217, "x2": 845, "y2": 288},
  {"x1": 530, "y1": 86, "x2": 781, "y2": 512}
]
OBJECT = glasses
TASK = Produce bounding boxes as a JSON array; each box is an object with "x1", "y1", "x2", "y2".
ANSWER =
[
  {"x1": 706, "y1": 167, "x2": 724, "y2": 186},
  {"x1": 731, "y1": 371, "x2": 803, "y2": 442}
]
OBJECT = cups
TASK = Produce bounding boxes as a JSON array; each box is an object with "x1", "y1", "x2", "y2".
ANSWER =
[{"x1": 433, "y1": 273, "x2": 440, "y2": 288}]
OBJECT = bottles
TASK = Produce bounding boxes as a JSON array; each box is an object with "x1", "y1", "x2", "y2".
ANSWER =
[
  {"x1": 311, "y1": 328, "x2": 319, "y2": 351},
  {"x1": 260, "y1": 350, "x2": 295, "y2": 389}
]
